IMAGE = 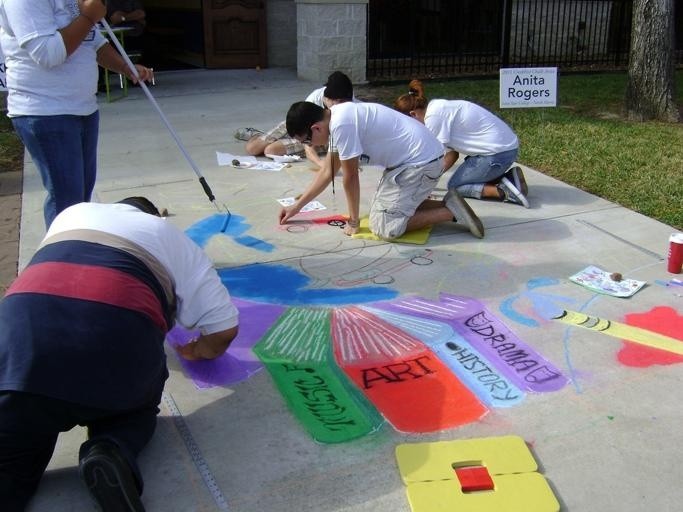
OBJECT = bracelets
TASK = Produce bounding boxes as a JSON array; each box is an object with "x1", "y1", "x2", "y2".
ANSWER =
[
  {"x1": 347, "y1": 218, "x2": 360, "y2": 228},
  {"x1": 78, "y1": 11, "x2": 96, "y2": 29}
]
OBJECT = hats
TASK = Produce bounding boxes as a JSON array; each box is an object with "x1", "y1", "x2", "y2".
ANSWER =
[{"x1": 324, "y1": 71, "x2": 353, "y2": 99}]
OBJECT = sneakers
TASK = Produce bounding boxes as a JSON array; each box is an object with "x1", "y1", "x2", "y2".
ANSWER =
[
  {"x1": 495, "y1": 177, "x2": 530, "y2": 208},
  {"x1": 444, "y1": 187, "x2": 484, "y2": 238},
  {"x1": 79, "y1": 444, "x2": 145, "y2": 512},
  {"x1": 234, "y1": 128, "x2": 263, "y2": 141},
  {"x1": 504, "y1": 167, "x2": 528, "y2": 198}
]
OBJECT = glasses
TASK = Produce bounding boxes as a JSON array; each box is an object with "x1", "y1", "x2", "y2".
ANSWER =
[{"x1": 301, "y1": 130, "x2": 312, "y2": 144}]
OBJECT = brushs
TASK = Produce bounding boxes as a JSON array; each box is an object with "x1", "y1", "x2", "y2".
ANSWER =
[{"x1": 100, "y1": 18, "x2": 231, "y2": 232}]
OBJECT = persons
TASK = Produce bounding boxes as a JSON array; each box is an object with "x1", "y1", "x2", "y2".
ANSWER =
[
  {"x1": 0, "y1": 1, "x2": 155, "y2": 236},
  {"x1": 393, "y1": 78, "x2": 530, "y2": 210},
  {"x1": 233, "y1": 69, "x2": 363, "y2": 161},
  {"x1": 277, "y1": 101, "x2": 485, "y2": 243},
  {"x1": 0, "y1": 194, "x2": 240, "y2": 511}
]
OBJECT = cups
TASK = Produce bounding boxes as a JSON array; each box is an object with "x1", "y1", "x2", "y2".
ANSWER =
[{"x1": 667, "y1": 232, "x2": 683, "y2": 274}]
